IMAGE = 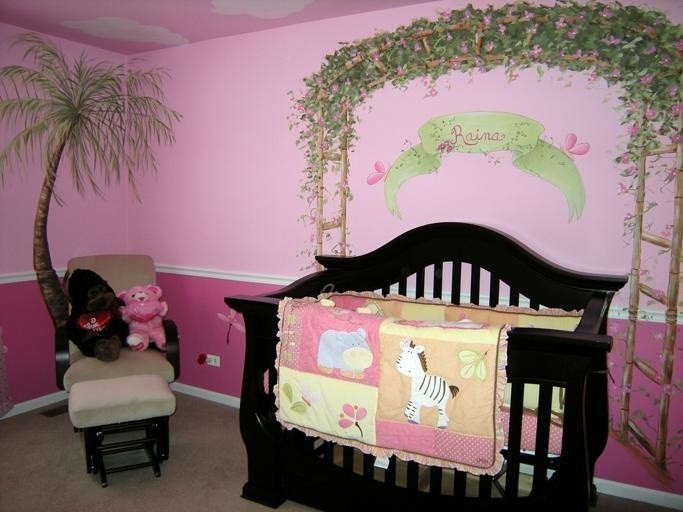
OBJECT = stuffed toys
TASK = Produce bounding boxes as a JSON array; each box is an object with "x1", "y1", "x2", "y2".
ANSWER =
[
  {"x1": 115, "y1": 284, "x2": 168, "y2": 352},
  {"x1": 65, "y1": 268, "x2": 127, "y2": 362}
]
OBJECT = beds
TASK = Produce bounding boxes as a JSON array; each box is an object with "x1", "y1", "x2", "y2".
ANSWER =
[{"x1": 223, "y1": 221, "x2": 628, "y2": 512}]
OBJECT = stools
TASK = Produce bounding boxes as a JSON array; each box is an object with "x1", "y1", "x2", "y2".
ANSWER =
[{"x1": 68, "y1": 375, "x2": 176, "y2": 488}]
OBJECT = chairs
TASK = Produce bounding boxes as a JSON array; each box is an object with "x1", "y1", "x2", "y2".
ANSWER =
[{"x1": 54, "y1": 254, "x2": 181, "y2": 472}]
